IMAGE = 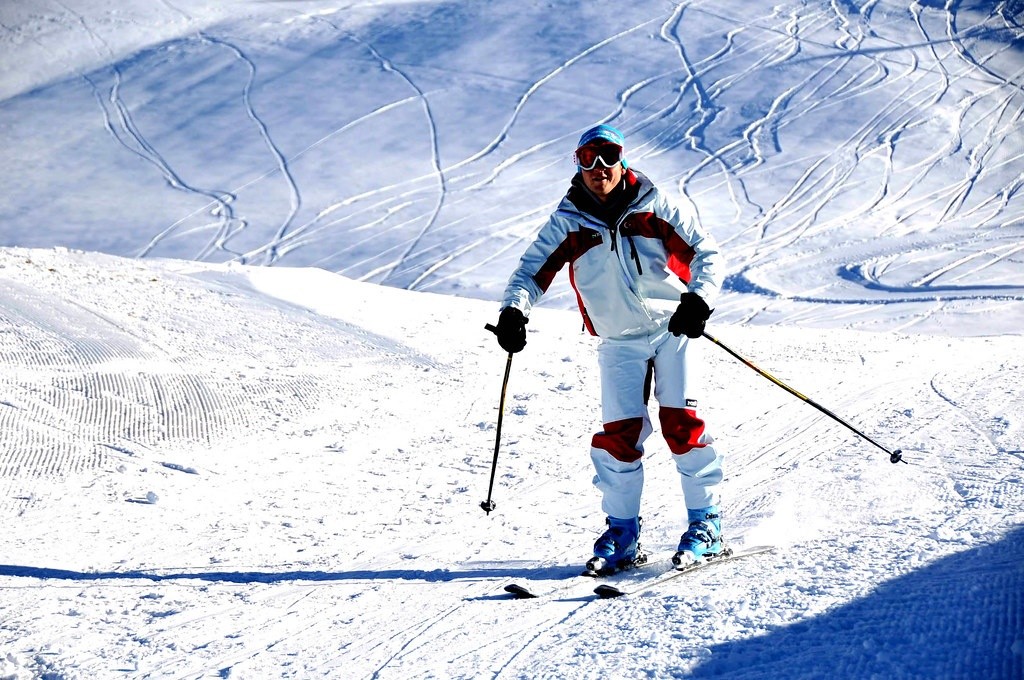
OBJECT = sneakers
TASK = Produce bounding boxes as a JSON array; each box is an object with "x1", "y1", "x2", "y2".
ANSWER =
[
  {"x1": 593, "y1": 516, "x2": 640, "y2": 562},
  {"x1": 678, "y1": 505, "x2": 720, "y2": 557}
]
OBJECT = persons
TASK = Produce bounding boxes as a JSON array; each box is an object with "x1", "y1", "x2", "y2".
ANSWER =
[{"x1": 486, "y1": 123, "x2": 730, "y2": 579}]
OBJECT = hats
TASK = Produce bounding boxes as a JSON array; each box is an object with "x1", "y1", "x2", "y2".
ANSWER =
[{"x1": 577, "y1": 124, "x2": 628, "y2": 169}]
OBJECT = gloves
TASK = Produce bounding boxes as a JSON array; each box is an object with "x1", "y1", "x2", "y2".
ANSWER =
[
  {"x1": 668, "y1": 292, "x2": 715, "y2": 339},
  {"x1": 484, "y1": 306, "x2": 529, "y2": 353}
]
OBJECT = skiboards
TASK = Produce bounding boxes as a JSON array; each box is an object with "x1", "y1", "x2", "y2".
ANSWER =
[{"x1": 503, "y1": 542, "x2": 777, "y2": 599}]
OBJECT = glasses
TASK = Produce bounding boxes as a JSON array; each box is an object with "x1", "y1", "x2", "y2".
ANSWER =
[{"x1": 573, "y1": 141, "x2": 624, "y2": 170}]
